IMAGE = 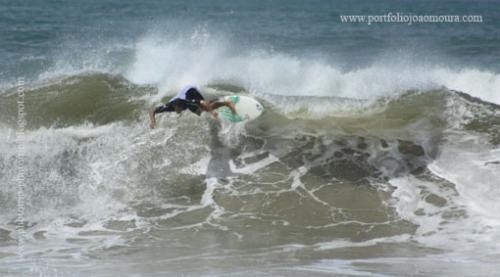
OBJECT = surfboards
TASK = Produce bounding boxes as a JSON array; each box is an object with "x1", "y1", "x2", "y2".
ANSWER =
[{"x1": 217, "y1": 96, "x2": 264, "y2": 122}]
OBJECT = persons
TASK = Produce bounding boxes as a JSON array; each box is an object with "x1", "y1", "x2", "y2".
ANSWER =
[{"x1": 148, "y1": 82, "x2": 239, "y2": 130}]
